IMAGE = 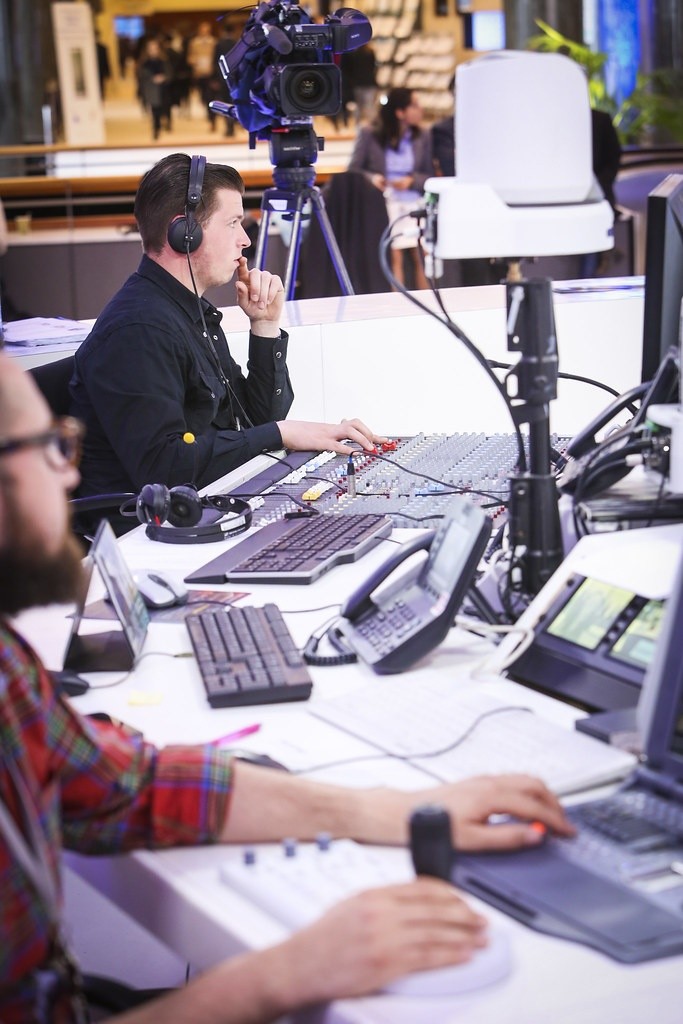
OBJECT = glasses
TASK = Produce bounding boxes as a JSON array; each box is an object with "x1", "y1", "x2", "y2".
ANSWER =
[{"x1": 0, "y1": 416, "x2": 80, "y2": 472}]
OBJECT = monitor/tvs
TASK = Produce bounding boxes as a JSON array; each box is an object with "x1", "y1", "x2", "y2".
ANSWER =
[{"x1": 642, "y1": 174, "x2": 683, "y2": 419}]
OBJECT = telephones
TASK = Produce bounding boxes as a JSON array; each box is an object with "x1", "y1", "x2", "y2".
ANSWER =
[{"x1": 327, "y1": 495, "x2": 494, "y2": 675}]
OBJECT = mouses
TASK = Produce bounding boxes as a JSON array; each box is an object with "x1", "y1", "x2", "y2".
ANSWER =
[{"x1": 104, "y1": 567, "x2": 189, "y2": 609}]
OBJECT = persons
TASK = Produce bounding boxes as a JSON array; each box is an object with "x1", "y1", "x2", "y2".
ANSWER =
[
  {"x1": 349, "y1": 88, "x2": 436, "y2": 292},
  {"x1": 64, "y1": 154, "x2": 389, "y2": 551},
  {"x1": 582, "y1": 108, "x2": 622, "y2": 278},
  {"x1": 0, "y1": 337, "x2": 577, "y2": 1024},
  {"x1": 432, "y1": 74, "x2": 522, "y2": 287},
  {"x1": 95, "y1": 30, "x2": 110, "y2": 99},
  {"x1": 132, "y1": 21, "x2": 238, "y2": 138}
]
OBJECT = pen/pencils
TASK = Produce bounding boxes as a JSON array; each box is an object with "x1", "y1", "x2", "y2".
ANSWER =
[{"x1": 213, "y1": 723, "x2": 261, "y2": 745}]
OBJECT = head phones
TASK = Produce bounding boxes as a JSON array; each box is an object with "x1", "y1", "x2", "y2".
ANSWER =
[
  {"x1": 168, "y1": 154, "x2": 206, "y2": 252},
  {"x1": 135, "y1": 483, "x2": 252, "y2": 544}
]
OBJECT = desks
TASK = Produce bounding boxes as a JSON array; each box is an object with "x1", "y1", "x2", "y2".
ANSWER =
[{"x1": 21, "y1": 449, "x2": 683, "y2": 1024}]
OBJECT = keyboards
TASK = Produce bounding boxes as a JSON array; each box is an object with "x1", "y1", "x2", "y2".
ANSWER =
[
  {"x1": 186, "y1": 514, "x2": 392, "y2": 584},
  {"x1": 186, "y1": 604, "x2": 312, "y2": 709}
]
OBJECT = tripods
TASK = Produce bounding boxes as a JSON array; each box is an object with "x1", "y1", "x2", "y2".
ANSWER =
[{"x1": 210, "y1": 101, "x2": 355, "y2": 303}]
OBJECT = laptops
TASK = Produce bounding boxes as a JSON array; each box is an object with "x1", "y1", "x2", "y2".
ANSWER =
[{"x1": 450, "y1": 562, "x2": 683, "y2": 964}]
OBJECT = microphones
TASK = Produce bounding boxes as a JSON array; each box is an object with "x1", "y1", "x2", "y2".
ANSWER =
[{"x1": 262, "y1": 22, "x2": 292, "y2": 53}]
